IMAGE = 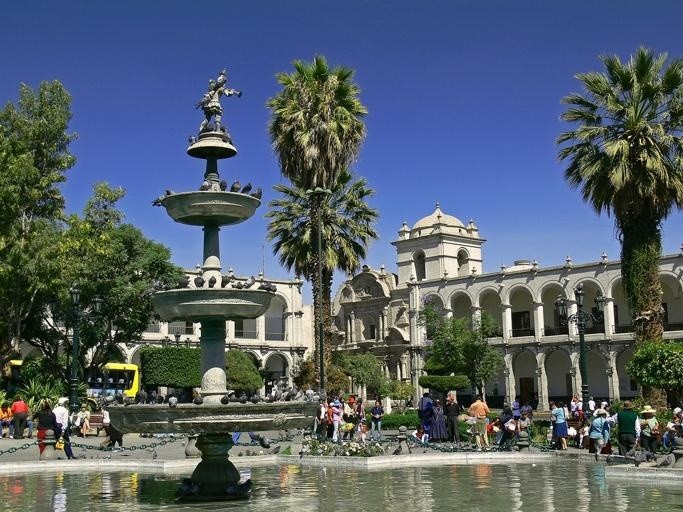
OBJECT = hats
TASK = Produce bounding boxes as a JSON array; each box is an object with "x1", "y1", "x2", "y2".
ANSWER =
[
  {"x1": 620, "y1": 400, "x2": 635, "y2": 409},
  {"x1": 673, "y1": 407, "x2": 681, "y2": 416},
  {"x1": 348, "y1": 398, "x2": 357, "y2": 404},
  {"x1": 58, "y1": 398, "x2": 68, "y2": 405},
  {"x1": 640, "y1": 406, "x2": 657, "y2": 413},
  {"x1": 596, "y1": 409, "x2": 608, "y2": 415}
]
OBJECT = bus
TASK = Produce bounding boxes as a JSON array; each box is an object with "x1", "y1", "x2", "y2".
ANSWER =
[{"x1": 4, "y1": 359, "x2": 140, "y2": 406}]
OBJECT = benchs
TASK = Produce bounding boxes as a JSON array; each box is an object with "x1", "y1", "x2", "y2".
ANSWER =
[
  {"x1": 74, "y1": 415, "x2": 104, "y2": 437},
  {"x1": 568, "y1": 420, "x2": 580, "y2": 445}
]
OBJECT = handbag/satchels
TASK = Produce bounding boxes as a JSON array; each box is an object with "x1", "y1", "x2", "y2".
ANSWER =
[{"x1": 56, "y1": 438, "x2": 64, "y2": 450}]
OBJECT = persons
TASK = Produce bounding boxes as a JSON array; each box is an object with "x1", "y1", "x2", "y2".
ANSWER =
[
  {"x1": 196, "y1": 68, "x2": 241, "y2": 132},
  {"x1": 0, "y1": 397, "x2": 122, "y2": 459},
  {"x1": 418, "y1": 393, "x2": 683, "y2": 457},
  {"x1": 313, "y1": 392, "x2": 385, "y2": 445}
]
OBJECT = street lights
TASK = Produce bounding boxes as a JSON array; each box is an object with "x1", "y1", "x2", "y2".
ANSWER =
[
  {"x1": 554, "y1": 281, "x2": 612, "y2": 418},
  {"x1": 304, "y1": 186, "x2": 332, "y2": 404},
  {"x1": 402, "y1": 346, "x2": 423, "y2": 389},
  {"x1": 45, "y1": 280, "x2": 106, "y2": 437},
  {"x1": 157, "y1": 332, "x2": 194, "y2": 349}
]
